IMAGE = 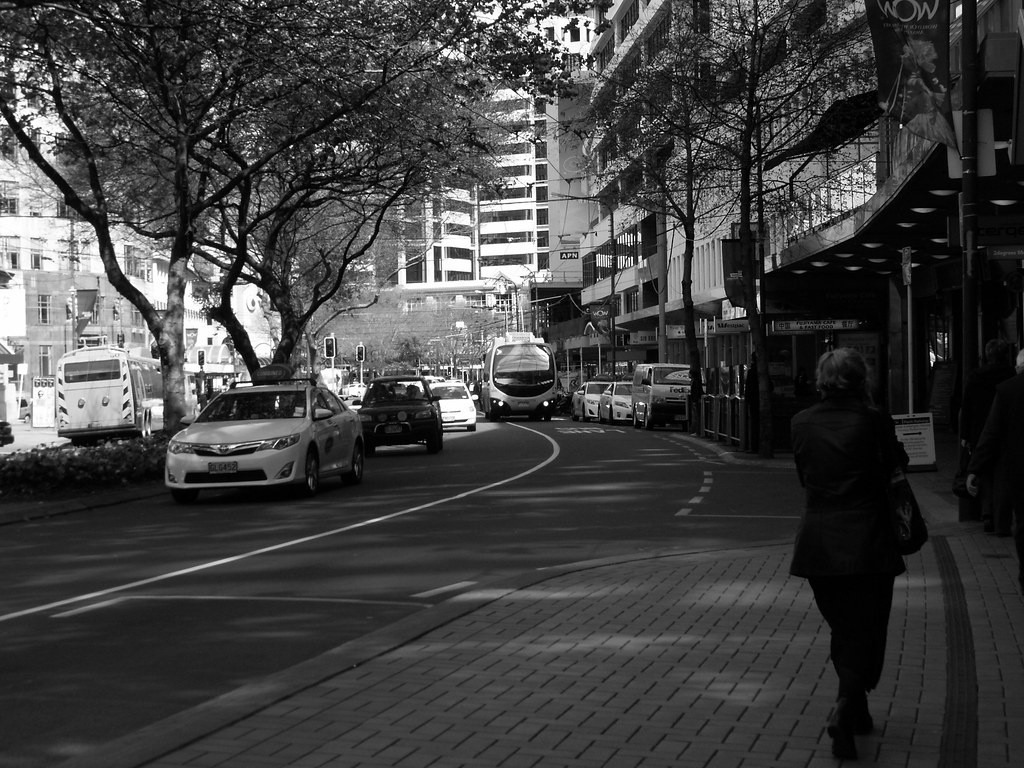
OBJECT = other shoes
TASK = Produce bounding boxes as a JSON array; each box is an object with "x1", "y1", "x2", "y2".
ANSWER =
[
  {"x1": 983, "y1": 515, "x2": 994, "y2": 532},
  {"x1": 852, "y1": 693, "x2": 873, "y2": 734},
  {"x1": 996, "y1": 527, "x2": 1013, "y2": 537},
  {"x1": 828, "y1": 697, "x2": 858, "y2": 761}
]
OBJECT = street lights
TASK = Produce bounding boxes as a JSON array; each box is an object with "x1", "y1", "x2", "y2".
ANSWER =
[{"x1": 551, "y1": 191, "x2": 616, "y2": 382}]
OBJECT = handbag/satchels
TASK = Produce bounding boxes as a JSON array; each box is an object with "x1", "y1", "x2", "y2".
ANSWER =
[
  {"x1": 952, "y1": 448, "x2": 985, "y2": 502},
  {"x1": 861, "y1": 403, "x2": 928, "y2": 556}
]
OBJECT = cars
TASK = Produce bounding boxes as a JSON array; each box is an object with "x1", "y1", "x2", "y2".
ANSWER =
[
  {"x1": 570, "y1": 381, "x2": 610, "y2": 423},
  {"x1": 598, "y1": 381, "x2": 634, "y2": 425},
  {"x1": 417, "y1": 381, "x2": 479, "y2": 432},
  {"x1": 328, "y1": 373, "x2": 478, "y2": 417},
  {"x1": 164, "y1": 363, "x2": 368, "y2": 505}
]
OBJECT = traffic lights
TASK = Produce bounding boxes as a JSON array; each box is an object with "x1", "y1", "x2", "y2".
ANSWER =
[
  {"x1": 324, "y1": 336, "x2": 336, "y2": 359},
  {"x1": 355, "y1": 345, "x2": 367, "y2": 363}
]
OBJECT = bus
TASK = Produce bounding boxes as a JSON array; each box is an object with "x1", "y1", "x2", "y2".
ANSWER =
[
  {"x1": 482, "y1": 331, "x2": 561, "y2": 423},
  {"x1": 53, "y1": 345, "x2": 163, "y2": 448}
]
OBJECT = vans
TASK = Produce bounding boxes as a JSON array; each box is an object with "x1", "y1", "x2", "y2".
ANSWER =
[{"x1": 631, "y1": 362, "x2": 706, "y2": 431}]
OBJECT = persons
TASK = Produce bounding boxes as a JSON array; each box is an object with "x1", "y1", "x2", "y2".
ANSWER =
[
  {"x1": 407, "y1": 385, "x2": 417, "y2": 398},
  {"x1": 790, "y1": 347, "x2": 909, "y2": 760},
  {"x1": 861, "y1": 368, "x2": 889, "y2": 409},
  {"x1": 958, "y1": 339, "x2": 1024, "y2": 589}
]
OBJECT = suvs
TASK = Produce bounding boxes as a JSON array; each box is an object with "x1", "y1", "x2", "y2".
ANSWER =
[{"x1": 352, "y1": 373, "x2": 442, "y2": 457}]
